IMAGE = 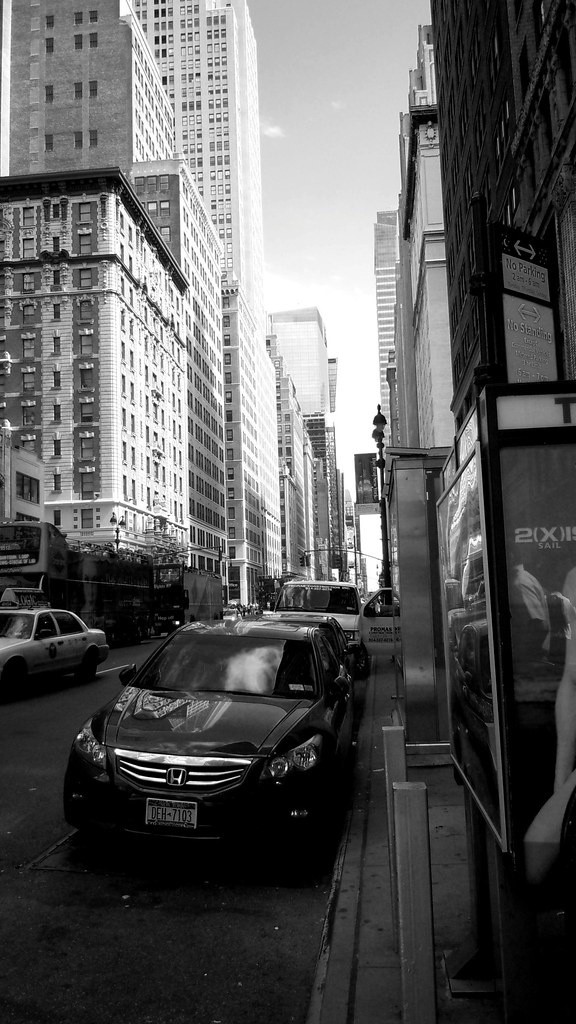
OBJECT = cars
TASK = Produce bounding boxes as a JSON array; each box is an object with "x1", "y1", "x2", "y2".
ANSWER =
[
  {"x1": 63, "y1": 613, "x2": 362, "y2": 848},
  {"x1": 0, "y1": 586, "x2": 110, "y2": 697}
]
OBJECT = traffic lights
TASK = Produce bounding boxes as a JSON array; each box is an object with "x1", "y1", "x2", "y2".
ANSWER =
[{"x1": 299, "y1": 556, "x2": 305, "y2": 567}]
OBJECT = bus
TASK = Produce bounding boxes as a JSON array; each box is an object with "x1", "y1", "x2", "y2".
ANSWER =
[{"x1": 1, "y1": 519, "x2": 225, "y2": 650}]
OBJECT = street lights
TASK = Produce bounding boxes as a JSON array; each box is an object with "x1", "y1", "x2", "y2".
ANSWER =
[
  {"x1": 370, "y1": 404, "x2": 394, "y2": 605},
  {"x1": 109, "y1": 511, "x2": 127, "y2": 553}
]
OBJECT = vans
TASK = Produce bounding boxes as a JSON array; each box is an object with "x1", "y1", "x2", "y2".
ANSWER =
[{"x1": 272, "y1": 581, "x2": 400, "y2": 681}]
